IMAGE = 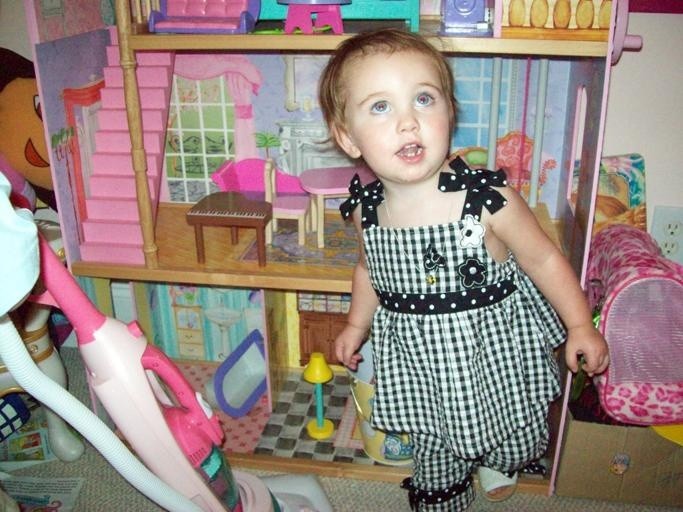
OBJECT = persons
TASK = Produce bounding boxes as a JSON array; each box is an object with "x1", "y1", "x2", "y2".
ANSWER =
[{"x1": 317, "y1": 28, "x2": 607, "y2": 512}]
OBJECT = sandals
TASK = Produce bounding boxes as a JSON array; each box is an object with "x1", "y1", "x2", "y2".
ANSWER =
[{"x1": 477, "y1": 465, "x2": 519, "y2": 502}]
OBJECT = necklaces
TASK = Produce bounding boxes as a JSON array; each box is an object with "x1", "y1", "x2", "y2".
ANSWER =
[{"x1": 379, "y1": 199, "x2": 457, "y2": 284}]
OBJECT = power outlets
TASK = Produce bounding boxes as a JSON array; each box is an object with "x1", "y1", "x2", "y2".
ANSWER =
[{"x1": 650, "y1": 206, "x2": 683, "y2": 266}]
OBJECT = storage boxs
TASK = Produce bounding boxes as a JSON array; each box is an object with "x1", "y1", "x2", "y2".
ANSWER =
[{"x1": 554, "y1": 390, "x2": 683, "y2": 509}]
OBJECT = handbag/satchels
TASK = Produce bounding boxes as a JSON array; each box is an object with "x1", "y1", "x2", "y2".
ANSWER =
[{"x1": 587, "y1": 224, "x2": 683, "y2": 426}]
